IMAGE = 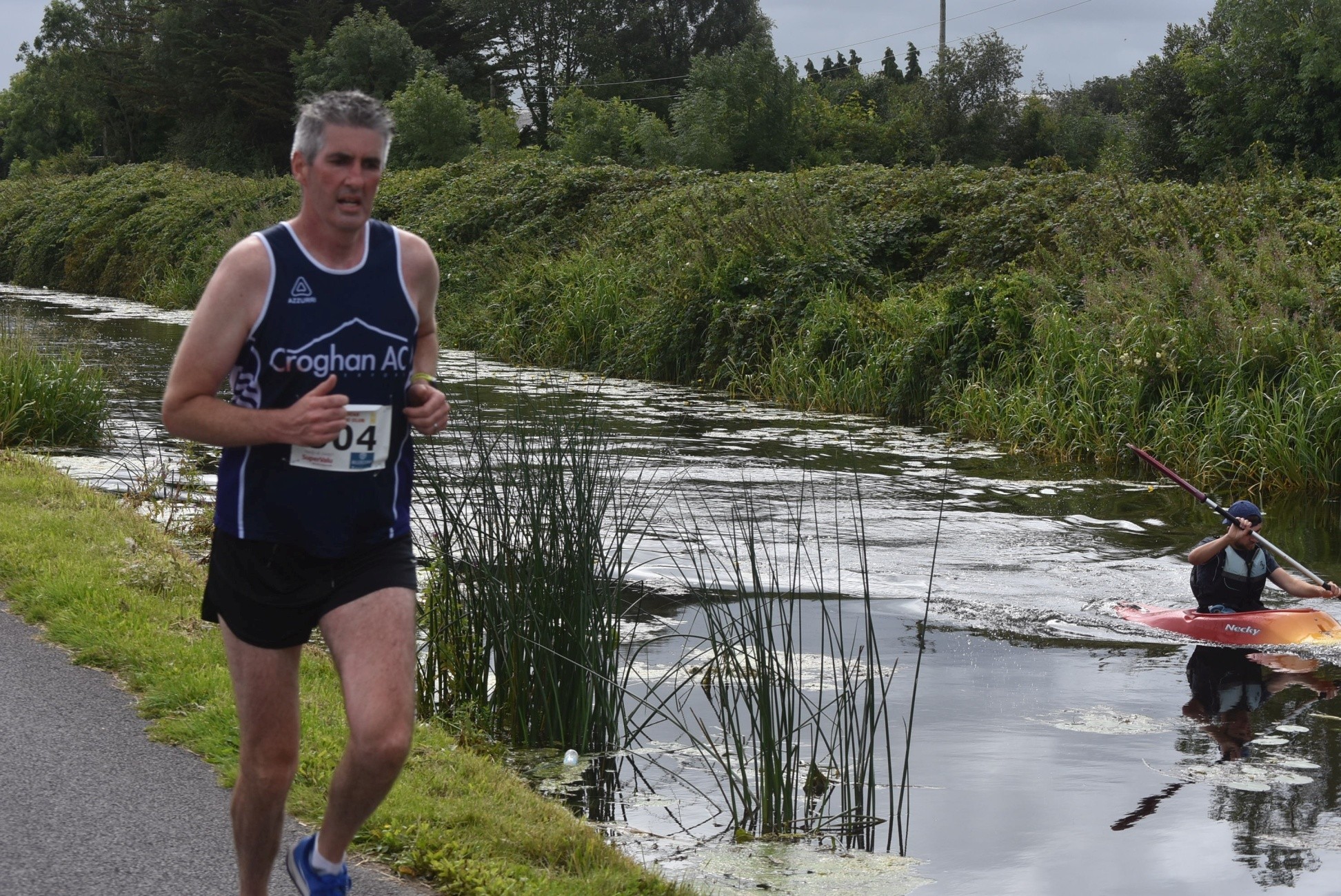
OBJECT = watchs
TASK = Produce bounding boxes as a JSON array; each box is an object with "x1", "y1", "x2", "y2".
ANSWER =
[{"x1": 412, "y1": 372, "x2": 437, "y2": 391}]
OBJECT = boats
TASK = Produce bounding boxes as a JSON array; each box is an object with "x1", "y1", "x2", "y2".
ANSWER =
[{"x1": 1113, "y1": 598, "x2": 1341, "y2": 652}]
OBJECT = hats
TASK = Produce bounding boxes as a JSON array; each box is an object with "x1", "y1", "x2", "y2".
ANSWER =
[{"x1": 1222, "y1": 500, "x2": 1262, "y2": 527}]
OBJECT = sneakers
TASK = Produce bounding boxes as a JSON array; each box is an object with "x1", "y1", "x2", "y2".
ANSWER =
[{"x1": 286, "y1": 831, "x2": 351, "y2": 896}]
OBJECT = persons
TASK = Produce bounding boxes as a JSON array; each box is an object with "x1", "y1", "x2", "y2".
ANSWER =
[
  {"x1": 160, "y1": 89, "x2": 452, "y2": 894},
  {"x1": 1187, "y1": 501, "x2": 1341, "y2": 613},
  {"x1": 1181, "y1": 646, "x2": 1339, "y2": 760}
]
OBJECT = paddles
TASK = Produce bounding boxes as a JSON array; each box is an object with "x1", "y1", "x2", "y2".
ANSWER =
[{"x1": 1121, "y1": 440, "x2": 1340, "y2": 598}]
